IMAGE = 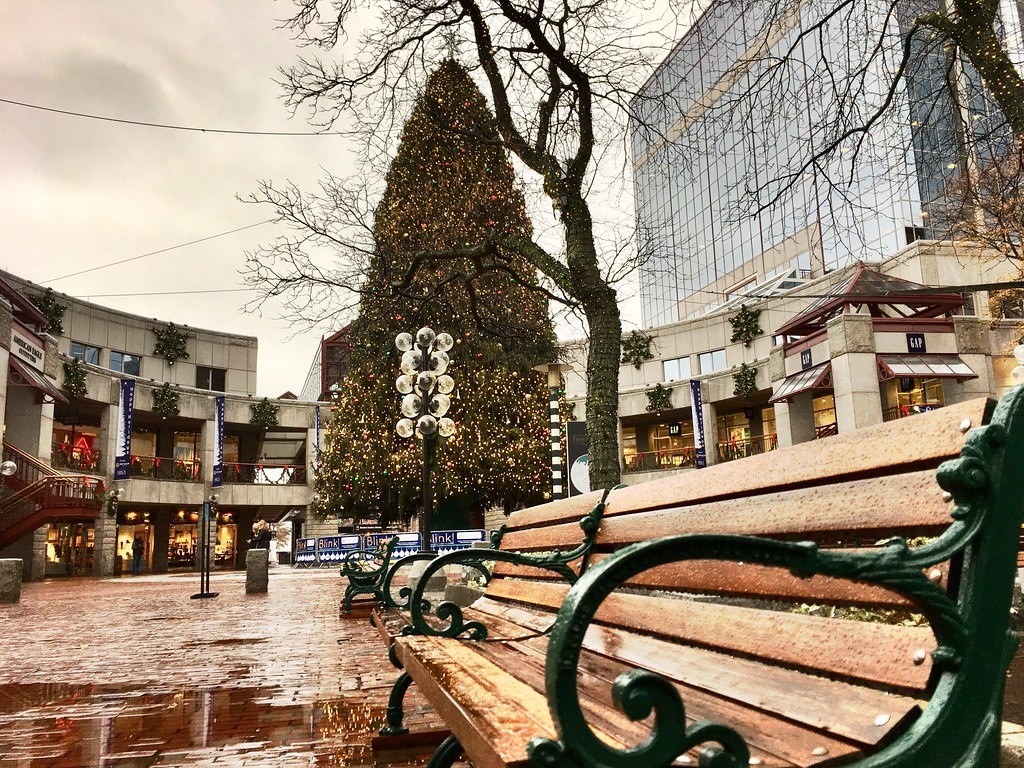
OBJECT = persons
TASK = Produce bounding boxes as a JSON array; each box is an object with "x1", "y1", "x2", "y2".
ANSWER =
[
  {"x1": 246, "y1": 523, "x2": 260, "y2": 549},
  {"x1": 252, "y1": 519, "x2": 272, "y2": 551},
  {"x1": 131, "y1": 536, "x2": 145, "y2": 575},
  {"x1": 171, "y1": 541, "x2": 176, "y2": 552}
]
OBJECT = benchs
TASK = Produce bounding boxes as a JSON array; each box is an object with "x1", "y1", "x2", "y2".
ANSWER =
[
  {"x1": 392, "y1": 381, "x2": 1024, "y2": 768},
  {"x1": 339, "y1": 536, "x2": 400, "y2": 614},
  {"x1": 368, "y1": 486, "x2": 615, "y2": 738}
]
